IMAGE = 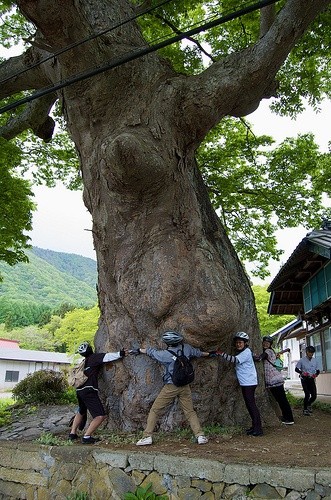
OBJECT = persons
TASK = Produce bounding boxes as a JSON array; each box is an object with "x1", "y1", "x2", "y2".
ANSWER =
[
  {"x1": 133, "y1": 332, "x2": 217, "y2": 446},
  {"x1": 216, "y1": 332, "x2": 264, "y2": 436},
  {"x1": 67, "y1": 343, "x2": 133, "y2": 444},
  {"x1": 259, "y1": 336, "x2": 294, "y2": 424},
  {"x1": 295, "y1": 346, "x2": 320, "y2": 416}
]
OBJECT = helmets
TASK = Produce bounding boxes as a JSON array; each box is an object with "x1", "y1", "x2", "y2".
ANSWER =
[
  {"x1": 306, "y1": 346, "x2": 315, "y2": 353},
  {"x1": 263, "y1": 336, "x2": 274, "y2": 345},
  {"x1": 162, "y1": 330, "x2": 184, "y2": 344},
  {"x1": 77, "y1": 342, "x2": 90, "y2": 353},
  {"x1": 235, "y1": 332, "x2": 250, "y2": 340}
]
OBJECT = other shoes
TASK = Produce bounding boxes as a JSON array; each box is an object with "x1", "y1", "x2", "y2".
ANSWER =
[
  {"x1": 280, "y1": 416, "x2": 294, "y2": 424},
  {"x1": 82, "y1": 436, "x2": 101, "y2": 444},
  {"x1": 68, "y1": 433, "x2": 79, "y2": 440},
  {"x1": 303, "y1": 409, "x2": 310, "y2": 416},
  {"x1": 308, "y1": 406, "x2": 313, "y2": 412}
]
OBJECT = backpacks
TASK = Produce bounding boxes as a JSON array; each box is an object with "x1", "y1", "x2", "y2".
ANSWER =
[
  {"x1": 163, "y1": 344, "x2": 195, "y2": 387},
  {"x1": 71, "y1": 359, "x2": 102, "y2": 389},
  {"x1": 264, "y1": 347, "x2": 283, "y2": 370}
]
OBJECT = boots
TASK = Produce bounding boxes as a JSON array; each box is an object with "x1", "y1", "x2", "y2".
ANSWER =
[{"x1": 246, "y1": 421, "x2": 263, "y2": 436}]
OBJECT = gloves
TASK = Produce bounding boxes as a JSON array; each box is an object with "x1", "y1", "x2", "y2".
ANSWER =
[
  {"x1": 208, "y1": 351, "x2": 223, "y2": 358},
  {"x1": 131, "y1": 348, "x2": 140, "y2": 355},
  {"x1": 302, "y1": 371, "x2": 316, "y2": 379}
]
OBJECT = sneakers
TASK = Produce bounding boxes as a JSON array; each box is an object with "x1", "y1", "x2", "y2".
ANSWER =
[
  {"x1": 197, "y1": 435, "x2": 208, "y2": 444},
  {"x1": 136, "y1": 436, "x2": 152, "y2": 446}
]
